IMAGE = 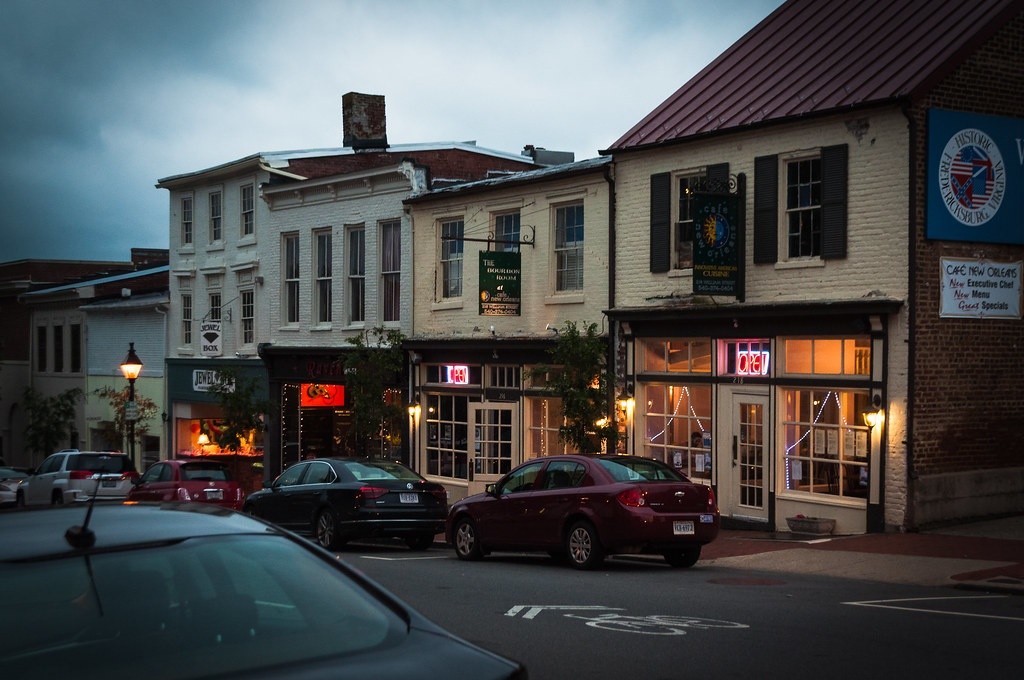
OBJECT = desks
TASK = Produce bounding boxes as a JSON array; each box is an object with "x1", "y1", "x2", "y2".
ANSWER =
[{"x1": 834, "y1": 476, "x2": 867, "y2": 482}]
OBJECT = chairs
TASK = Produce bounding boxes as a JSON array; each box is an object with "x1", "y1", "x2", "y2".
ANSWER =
[
  {"x1": 826, "y1": 458, "x2": 866, "y2": 497},
  {"x1": 193, "y1": 592, "x2": 258, "y2": 645},
  {"x1": 551, "y1": 470, "x2": 575, "y2": 488}
]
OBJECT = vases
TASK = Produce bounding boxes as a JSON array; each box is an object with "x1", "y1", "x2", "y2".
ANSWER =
[{"x1": 786, "y1": 517, "x2": 835, "y2": 535}]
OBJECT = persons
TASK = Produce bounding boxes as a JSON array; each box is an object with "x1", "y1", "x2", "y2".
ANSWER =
[{"x1": 682, "y1": 432, "x2": 703, "y2": 466}]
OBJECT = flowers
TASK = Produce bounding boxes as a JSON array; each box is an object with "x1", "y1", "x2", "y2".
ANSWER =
[{"x1": 797, "y1": 514, "x2": 805, "y2": 519}]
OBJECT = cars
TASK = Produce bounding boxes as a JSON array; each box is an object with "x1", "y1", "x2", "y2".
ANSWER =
[
  {"x1": 0, "y1": 466, "x2": 29, "y2": 506},
  {"x1": 0, "y1": 503, "x2": 532, "y2": 680},
  {"x1": 240, "y1": 456, "x2": 450, "y2": 554},
  {"x1": 122, "y1": 455, "x2": 244, "y2": 513},
  {"x1": 444, "y1": 454, "x2": 720, "y2": 571}
]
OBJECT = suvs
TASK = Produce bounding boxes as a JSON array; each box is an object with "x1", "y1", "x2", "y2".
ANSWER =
[{"x1": 15, "y1": 449, "x2": 142, "y2": 509}]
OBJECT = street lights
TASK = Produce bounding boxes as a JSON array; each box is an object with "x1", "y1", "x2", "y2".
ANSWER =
[{"x1": 121, "y1": 341, "x2": 143, "y2": 472}]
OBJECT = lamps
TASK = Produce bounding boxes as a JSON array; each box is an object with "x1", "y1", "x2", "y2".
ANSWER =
[
  {"x1": 491, "y1": 325, "x2": 496, "y2": 337},
  {"x1": 545, "y1": 324, "x2": 558, "y2": 335},
  {"x1": 408, "y1": 398, "x2": 417, "y2": 415},
  {"x1": 235, "y1": 352, "x2": 248, "y2": 358},
  {"x1": 198, "y1": 433, "x2": 210, "y2": 456},
  {"x1": 860, "y1": 394, "x2": 881, "y2": 432},
  {"x1": 492, "y1": 350, "x2": 498, "y2": 358}
]
